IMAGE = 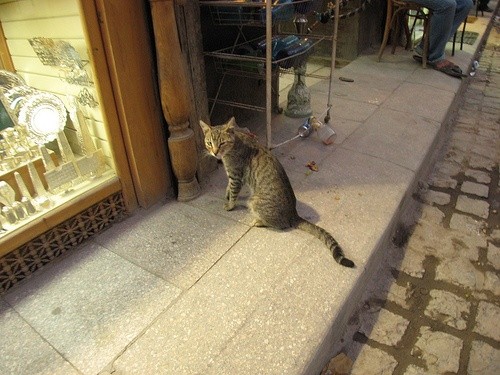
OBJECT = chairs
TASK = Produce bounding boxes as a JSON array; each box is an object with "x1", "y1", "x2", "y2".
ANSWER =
[
  {"x1": 378, "y1": 0, "x2": 436, "y2": 69},
  {"x1": 405, "y1": 5, "x2": 468, "y2": 57}
]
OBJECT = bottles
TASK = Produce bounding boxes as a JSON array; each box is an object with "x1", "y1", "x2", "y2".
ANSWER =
[{"x1": 308, "y1": 117, "x2": 337, "y2": 144}]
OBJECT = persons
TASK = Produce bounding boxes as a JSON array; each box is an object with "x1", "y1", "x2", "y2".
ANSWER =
[{"x1": 404, "y1": 0, "x2": 476, "y2": 78}]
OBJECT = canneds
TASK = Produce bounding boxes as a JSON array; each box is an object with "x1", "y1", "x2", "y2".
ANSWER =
[
  {"x1": 298, "y1": 119, "x2": 313, "y2": 137},
  {"x1": 259, "y1": 35, "x2": 309, "y2": 62}
]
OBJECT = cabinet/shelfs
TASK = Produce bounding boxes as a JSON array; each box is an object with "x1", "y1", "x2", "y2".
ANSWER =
[{"x1": 201, "y1": 0, "x2": 340, "y2": 156}]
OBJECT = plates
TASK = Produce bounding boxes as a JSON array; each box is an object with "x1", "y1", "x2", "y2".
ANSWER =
[{"x1": 1, "y1": 71, "x2": 68, "y2": 145}]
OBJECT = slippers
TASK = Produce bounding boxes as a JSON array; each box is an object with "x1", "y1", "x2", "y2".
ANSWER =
[{"x1": 413, "y1": 54, "x2": 467, "y2": 79}]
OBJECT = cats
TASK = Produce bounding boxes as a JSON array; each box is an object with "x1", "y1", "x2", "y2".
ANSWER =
[{"x1": 199, "y1": 116, "x2": 355, "y2": 267}]
